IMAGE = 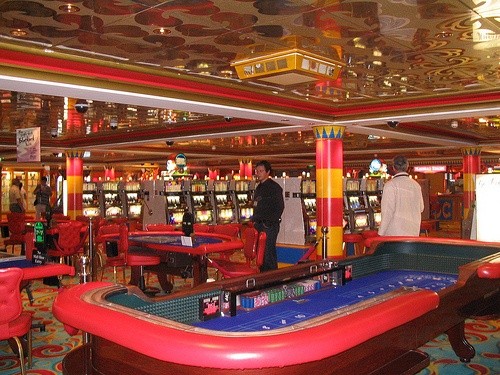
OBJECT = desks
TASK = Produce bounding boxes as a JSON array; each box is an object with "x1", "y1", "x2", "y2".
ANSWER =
[
  {"x1": 0, "y1": 257, "x2": 75, "y2": 334},
  {"x1": 96, "y1": 224, "x2": 243, "y2": 292},
  {"x1": 54, "y1": 233, "x2": 500, "y2": 375},
  {"x1": 0, "y1": 216, "x2": 89, "y2": 255}
]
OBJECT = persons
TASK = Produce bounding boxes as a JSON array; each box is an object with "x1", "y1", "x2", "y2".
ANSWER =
[
  {"x1": 377, "y1": 155, "x2": 423, "y2": 236},
  {"x1": 250, "y1": 161, "x2": 286, "y2": 273},
  {"x1": 8, "y1": 177, "x2": 53, "y2": 218}
]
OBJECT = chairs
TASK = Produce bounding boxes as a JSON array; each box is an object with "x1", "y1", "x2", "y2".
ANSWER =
[{"x1": 0, "y1": 210, "x2": 438, "y2": 375}]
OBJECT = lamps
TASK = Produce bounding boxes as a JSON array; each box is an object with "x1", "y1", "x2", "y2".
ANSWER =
[
  {"x1": 369, "y1": 157, "x2": 388, "y2": 176},
  {"x1": 175, "y1": 152, "x2": 188, "y2": 176},
  {"x1": 228, "y1": 51, "x2": 346, "y2": 93}
]
